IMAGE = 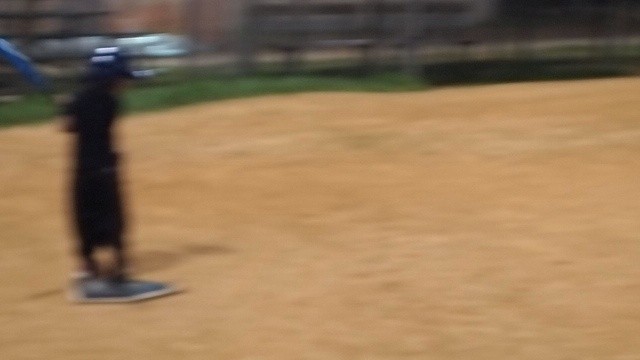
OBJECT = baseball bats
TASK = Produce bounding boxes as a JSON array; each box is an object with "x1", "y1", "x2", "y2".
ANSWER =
[{"x1": 0, "y1": 38, "x2": 65, "y2": 110}]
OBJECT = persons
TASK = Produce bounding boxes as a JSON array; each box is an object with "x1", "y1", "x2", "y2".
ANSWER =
[{"x1": 49, "y1": 44, "x2": 165, "y2": 287}]
